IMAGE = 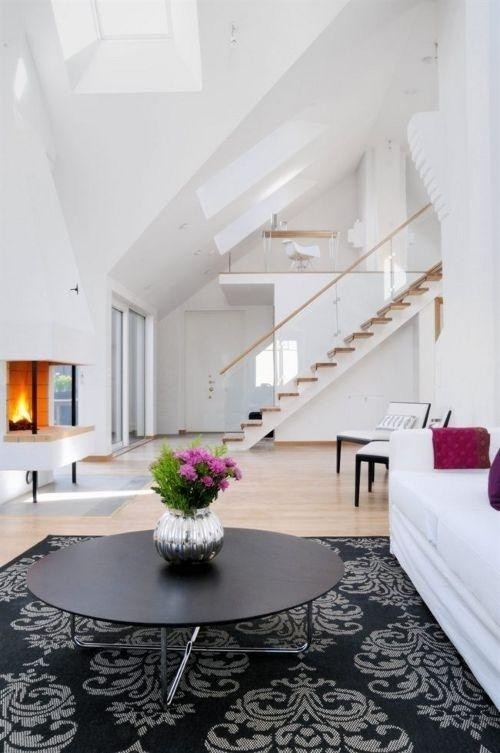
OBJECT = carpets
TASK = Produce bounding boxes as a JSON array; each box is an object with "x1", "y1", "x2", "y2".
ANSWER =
[{"x1": 1, "y1": 533, "x2": 499, "y2": 752}]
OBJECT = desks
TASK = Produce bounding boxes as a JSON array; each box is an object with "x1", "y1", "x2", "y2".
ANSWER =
[
  {"x1": 25, "y1": 524, "x2": 348, "y2": 705},
  {"x1": 260, "y1": 228, "x2": 340, "y2": 274}
]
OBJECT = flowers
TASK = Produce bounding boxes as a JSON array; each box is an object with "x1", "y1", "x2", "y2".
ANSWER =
[{"x1": 146, "y1": 427, "x2": 243, "y2": 521}]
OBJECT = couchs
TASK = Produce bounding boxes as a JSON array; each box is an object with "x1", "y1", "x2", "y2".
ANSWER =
[{"x1": 383, "y1": 422, "x2": 498, "y2": 719}]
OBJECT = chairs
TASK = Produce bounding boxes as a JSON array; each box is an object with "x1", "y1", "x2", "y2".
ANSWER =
[
  {"x1": 335, "y1": 402, "x2": 432, "y2": 481},
  {"x1": 350, "y1": 408, "x2": 455, "y2": 505}
]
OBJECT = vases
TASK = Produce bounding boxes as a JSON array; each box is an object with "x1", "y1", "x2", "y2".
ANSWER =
[{"x1": 153, "y1": 505, "x2": 223, "y2": 564}]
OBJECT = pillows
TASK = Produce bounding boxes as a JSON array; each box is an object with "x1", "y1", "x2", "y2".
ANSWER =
[
  {"x1": 373, "y1": 413, "x2": 419, "y2": 430},
  {"x1": 487, "y1": 447, "x2": 499, "y2": 510}
]
